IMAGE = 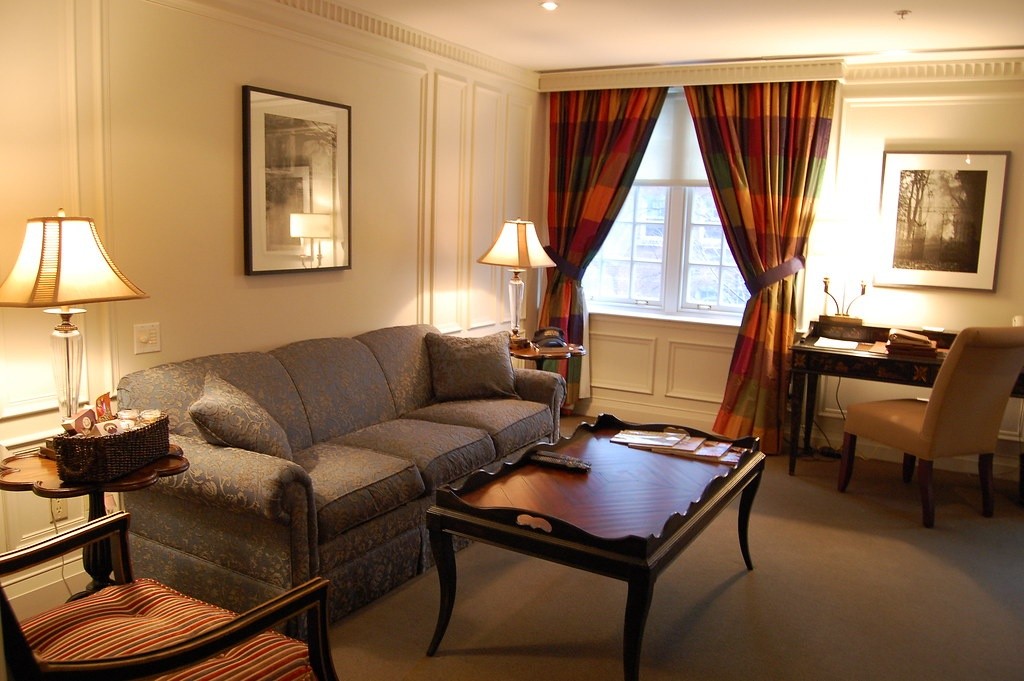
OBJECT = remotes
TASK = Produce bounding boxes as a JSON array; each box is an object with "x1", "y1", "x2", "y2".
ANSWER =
[{"x1": 530, "y1": 450, "x2": 592, "y2": 472}]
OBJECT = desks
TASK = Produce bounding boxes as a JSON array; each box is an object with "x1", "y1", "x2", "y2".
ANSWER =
[{"x1": 786, "y1": 331, "x2": 1024, "y2": 476}]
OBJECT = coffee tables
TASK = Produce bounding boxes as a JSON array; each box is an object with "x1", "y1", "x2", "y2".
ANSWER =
[{"x1": 428, "y1": 413, "x2": 767, "y2": 680}]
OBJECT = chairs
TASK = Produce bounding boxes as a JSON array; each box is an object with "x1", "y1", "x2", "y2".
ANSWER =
[
  {"x1": 0, "y1": 510, "x2": 338, "y2": 681},
  {"x1": 835, "y1": 325, "x2": 1024, "y2": 527}
]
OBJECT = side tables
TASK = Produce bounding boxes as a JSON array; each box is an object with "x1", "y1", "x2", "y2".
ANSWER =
[
  {"x1": 0, "y1": 444, "x2": 190, "y2": 600},
  {"x1": 508, "y1": 343, "x2": 586, "y2": 404}
]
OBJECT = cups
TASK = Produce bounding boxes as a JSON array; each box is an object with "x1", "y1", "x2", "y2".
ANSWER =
[{"x1": 117, "y1": 410, "x2": 161, "y2": 429}]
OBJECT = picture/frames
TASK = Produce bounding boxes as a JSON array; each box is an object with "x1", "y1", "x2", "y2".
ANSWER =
[
  {"x1": 874, "y1": 151, "x2": 1010, "y2": 292},
  {"x1": 242, "y1": 84, "x2": 355, "y2": 277}
]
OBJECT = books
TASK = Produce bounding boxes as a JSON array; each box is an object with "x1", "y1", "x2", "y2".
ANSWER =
[
  {"x1": 869, "y1": 327, "x2": 937, "y2": 360},
  {"x1": 610, "y1": 426, "x2": 746, "y2": 467}
]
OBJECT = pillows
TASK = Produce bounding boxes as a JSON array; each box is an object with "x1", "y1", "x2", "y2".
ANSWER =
[
  {"x1": 187, "y1": 367, "x2": 292, "y2": 467},
  {"x1": 420, "y1": 329, "x2": 514, "y2": 401}
]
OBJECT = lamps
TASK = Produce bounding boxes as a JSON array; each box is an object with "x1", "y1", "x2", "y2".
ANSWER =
[
  {"x1": 0, "y1": 208, "x2": 151, "y2": 458},
  {"x1": 479, "y1": 218, "x2": 556, "y2": 347},
  {"x1": 819, "y1": 276, "x2": 867, "y2": 326}
]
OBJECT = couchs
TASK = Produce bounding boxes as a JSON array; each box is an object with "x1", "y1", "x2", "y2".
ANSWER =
[{"x1": 112, "y1": 325, "x2": 563, "y2": 633}]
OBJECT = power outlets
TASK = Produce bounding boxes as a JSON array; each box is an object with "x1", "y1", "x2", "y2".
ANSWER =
[{"x1": 50, "y1": 497, "x2": 68, "y2": 523}]
